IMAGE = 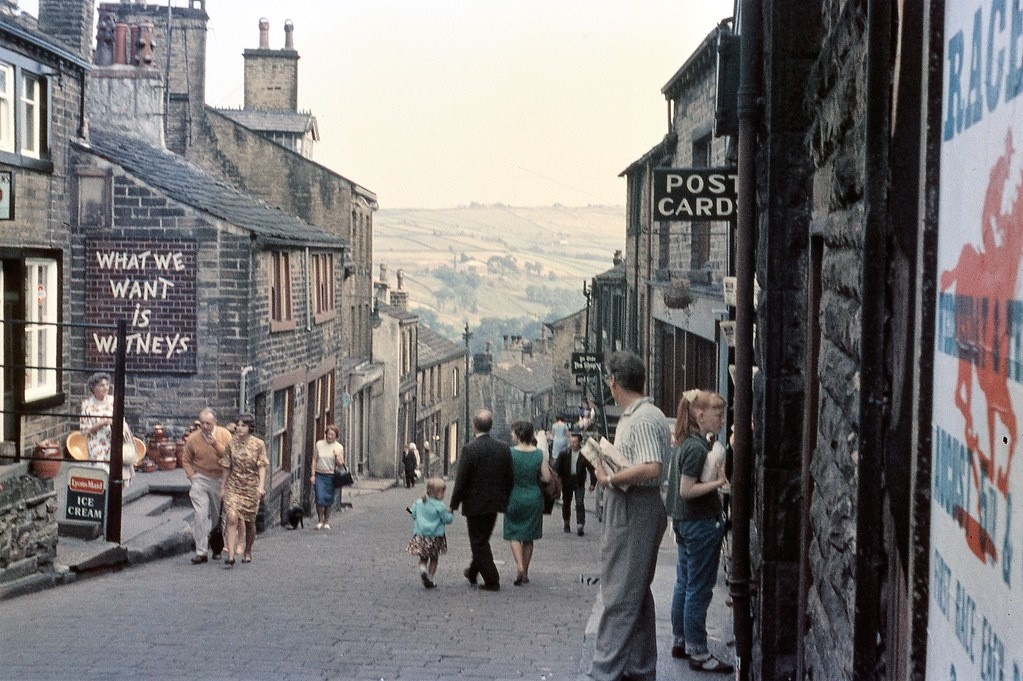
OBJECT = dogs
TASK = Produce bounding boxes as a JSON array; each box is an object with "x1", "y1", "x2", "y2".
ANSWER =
[{"x1": 288, "y1": 505, "x2": 304, "y2": 529}]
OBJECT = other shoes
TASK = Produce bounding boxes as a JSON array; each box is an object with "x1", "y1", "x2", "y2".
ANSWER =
[
  {"x1": 191, "y1": 556, "x2": 208, "y2": 563},
  {"x1": 673, "y1": 646, "x2": 689, "y2": 658},
  {"x1": 463, "y1": 568, "x2": 476, "y2": 583},
  {"x1": 480, "y1": 583, "x2": 500, "y2": 591},
  {"x1": 212, "y1": 553, "x2": 222, "y2": 559},
  {"x1": 578, "y1": 530, "x2": 584, "y2": 536},
  {"x1": 688, "y1": 654, "x2": 733, "y2": 673},
  {"x1": 420, "y1": 571, "x2": 433, "y2": 587},
  {"x1": 563, "y1": 523, "x2": 570, "y2": 532},
  {"x1": 315, "y1": 522, "x2": 322, "y2": 529},
  {"x1": 324, "y1": 523, "x2": 329, "y2": 530},
  {"x1": 514, "y1": 571, "x2": 524, "y2": 585},
  {"x1": 225, "y1": 558, "x2": 235, "y2": 565}
]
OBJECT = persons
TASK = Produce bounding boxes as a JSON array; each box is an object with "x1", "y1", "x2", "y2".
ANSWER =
[
  {"x1": 665, "y1": 390, "x2": 734, "y2": 674},
  {"x1": 448, "y1": 410, "x2": 515, "y2": 591},
  {"x1": 181, "y1": 407, "x2": 232, "y2": 563},
  {"x1": 405, "y1": 478, "x2": 454, "y2": 589},
  {"x1": 310, "y1": 425, "x2": 344, "y2": 530},
  {"x1": 535, "y1": 424, "x2": 550, "y2": 462},
  {"x1": 80, "y1": 372, "x2": 135, "y2": 488},
  {"x1": 403, "y1": 446, "x2": 416, "y2": 488},
  {"x1": 589, "y1": 351, "x2": 671, "y2": 681},
  {"x1": 218, "y1": 414, "x2": 269, "y2": 565},
  {"x1": 503, "y1": 422, "x2": 551, "y2": 585},
  {"x1": 552, "y1": 415, "x2": 570, "y2": 463},
  {"x1": 553, "y1": 433, "x2": 597, "y2": 536},
  {"x1": 409, "y1": 443, "x2": 421, "y2": 481}
]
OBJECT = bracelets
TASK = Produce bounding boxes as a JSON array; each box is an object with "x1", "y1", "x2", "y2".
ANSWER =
[{"x1": 605, "y1": 476, "x2": 614, "y2": 490}]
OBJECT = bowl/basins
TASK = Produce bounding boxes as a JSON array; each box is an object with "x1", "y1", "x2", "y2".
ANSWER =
[
  {"x1": 132, "y1": 436, "x2": 146, "y2": 461},
  {"x1": 66, "y1": 432, "x2": 89, "y2": 460}
]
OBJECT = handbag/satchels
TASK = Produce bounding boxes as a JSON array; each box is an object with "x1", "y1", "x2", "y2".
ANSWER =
[
  {"x1": 333, "y1": 463, "x2": 354, "y2": 488},
  {"x1": 208, "y1": 525, "x2": 224, "y2": 553}
]
